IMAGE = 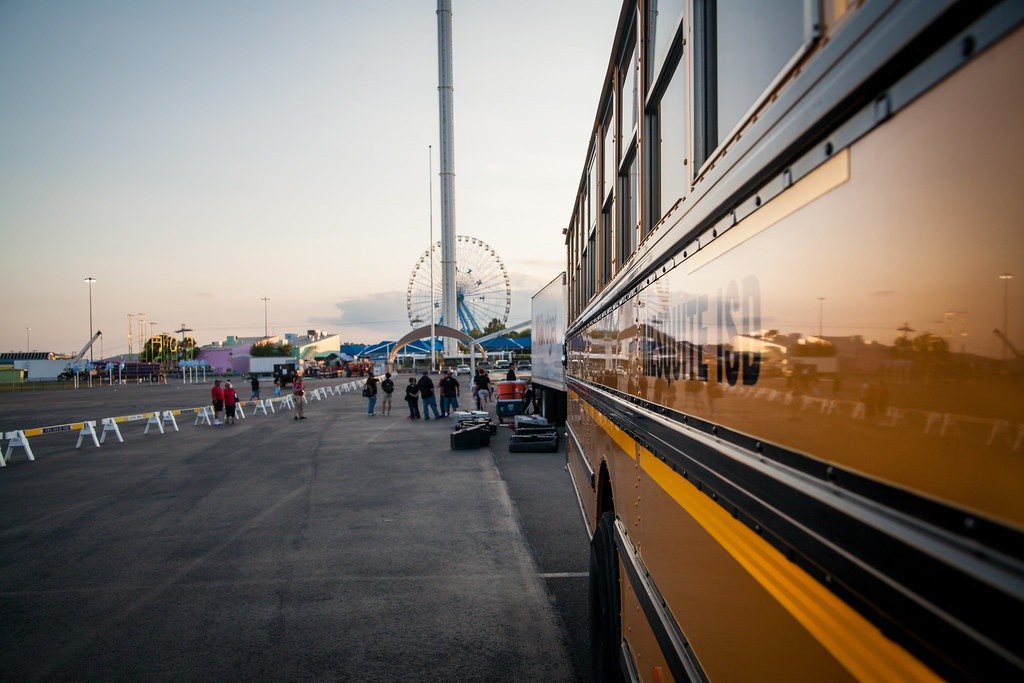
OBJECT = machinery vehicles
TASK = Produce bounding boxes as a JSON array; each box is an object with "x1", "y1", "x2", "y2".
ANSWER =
[{"x1": 57, "y1": 330, "x2": 103, "y2": 382}]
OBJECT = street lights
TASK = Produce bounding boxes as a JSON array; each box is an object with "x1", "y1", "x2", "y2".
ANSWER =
[
  {"x1": 85, "y1": 278, "x2": 96, "y2": 361},
  {"x1": 999, "y1": 273, "x2": 1013, "y2": 360},
  {"x1": 27, "y1": 328, "x2": 31, "y2": 352},
  {"x1": 261, "y1": 297, "x2": 269, "y2": 336},
  {"x1": 818, "y1": 297, "x2": 825, "y2": 337},
  {"x1": 150, "y1": 323, "x2": 157, "y2": 362}
]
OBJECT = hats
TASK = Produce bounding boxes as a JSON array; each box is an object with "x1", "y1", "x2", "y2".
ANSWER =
[{"x1": 214, "y1": 380, "x2": 222, "y2": 382}]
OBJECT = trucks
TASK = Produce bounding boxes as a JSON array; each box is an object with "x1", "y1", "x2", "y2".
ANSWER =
[{"x1": 273, "y1": 363, "x2": 302, "y2": 388}]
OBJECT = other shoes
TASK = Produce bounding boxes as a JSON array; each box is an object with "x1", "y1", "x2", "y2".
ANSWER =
[
  {"x1": 424, "y1": 417, "x2": 430, "y2": 420},
  {"x1": 408, "y1": 416, "x2": 421, "y2": 419},
  {"x1": 294, "y1": 416, "x2": 299, "y2": 420},
  {"x1": 225, "y1": 421, "x2": 230, "y2": 424},
  {"x1": 300, "y1": 417, "x2": 307, "y2": 419},
  {"x1": 441, "y1": 415, "x2": 449, "y2": 417},
  {"x1": 214, "y1": 422, "x2": 222, "y2": 426},
  {"x1": 436, "y1": 416, "x2": 442, "y2": 419},
  {"x1": 369, "y1": 413, "x2": 377, "y2": 416}
]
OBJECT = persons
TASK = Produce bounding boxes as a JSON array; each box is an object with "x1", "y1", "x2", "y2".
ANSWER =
[
  {"x1": 273, "y1": 372, "x2": 283, "y2": 396},
  {"x1": 439, "y1": 370, "x2": 460, "y2": 416},
  {"x1": 507, "y1": 363, "x2": 516, "y2": 380},
  {"x1": 224, "y1": 382, "x2": 236, "y2": 424},
  {"x1": 418, "y1": 370, "x2": 442, "y2": 419},
  {"x1": 227, "y1": 379, "x2": 233, "y2": 388},
  {"x1": 292, "y1": 376, "x2": 307, "y2": 419},
  {"x1": 437, "y1": 370, "x2": 449, "y2": 416},
  {"x1": 212, "y1": 380, "x2": 224, "y2": 425},
  {"x1": 367, "y1": 373, "x2": 380, "y2": 416},
  {"x1": 473, "y1": 368, "x2": 493, "y2": 412},
  {"x1": 405, "y1": 377, "x2": 421, "y2": 419},
  {"x1": 381, "y1": 372, "x2": 394, "y2": 416},
  {"x1": 250, "y1": 375, "x2": 260, "y2": 400}
]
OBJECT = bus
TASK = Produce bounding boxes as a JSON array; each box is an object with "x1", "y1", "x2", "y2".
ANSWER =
[{"x1": 562, "y1": 1, "x2": 1023, "y2": 683}]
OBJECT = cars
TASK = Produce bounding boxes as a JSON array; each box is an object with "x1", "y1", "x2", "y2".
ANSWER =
[
  {"x1": 477, "y1": 362, "x2": 493, "y2": 370},
  {"x1": 786, "y1": 363, "x2": 819, "y2": 388},
  {"x1": 493, "y1": 360, "x2": 509, "y2": 369},
  {"x1": 457, "y1": 365, "x2": 470, "y2": 375},
  {"x1": 516, "y1": 360, "x2": 531, "y2": 370}
]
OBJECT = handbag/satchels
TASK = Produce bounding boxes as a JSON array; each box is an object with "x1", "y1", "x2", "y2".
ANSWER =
[
  {"x1": 362, "y1": 390, "x2": 374, "y2": 397},
  {"x1": 234, "y1": 393, "x2": 239, "y2": 402}
]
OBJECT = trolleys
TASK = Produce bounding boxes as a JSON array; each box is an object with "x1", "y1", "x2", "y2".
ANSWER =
[{"x1": 495, "y1": 393, "x2": 529, "y2": 422}]
